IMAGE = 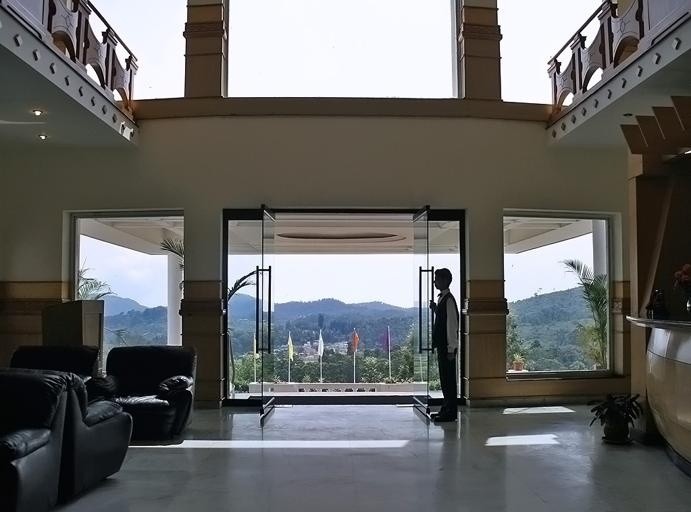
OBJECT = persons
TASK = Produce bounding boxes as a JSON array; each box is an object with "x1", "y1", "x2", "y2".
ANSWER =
[{"x1": 430, "y1": 268, "x2": 460, "y2": 422}]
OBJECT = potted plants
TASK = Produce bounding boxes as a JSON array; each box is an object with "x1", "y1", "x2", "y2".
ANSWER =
[
  {"x1": 587, "y1": 393, "x2": 643, "y2": 443},
  {"x1": 234, "y1": 382, "x2": 241, "y2": 393},
  {"x1": 241, "y1": 382, "x2": 249, "y2": 393},
  {"x1": 565, "y1": 261, "x2": 607, "y2": 370},
  {"x1": 513, "y1": 353, "x2": 526, "y2": 371}
]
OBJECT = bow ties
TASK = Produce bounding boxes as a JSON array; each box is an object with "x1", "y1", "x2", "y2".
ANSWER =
[{"x1": 437, "y1": 293, "x2": 443, "y2": 298}]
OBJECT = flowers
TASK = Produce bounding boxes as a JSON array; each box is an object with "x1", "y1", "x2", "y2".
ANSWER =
[{"x1": 671, "y1": 264, "x2": 691, "y2": 296}]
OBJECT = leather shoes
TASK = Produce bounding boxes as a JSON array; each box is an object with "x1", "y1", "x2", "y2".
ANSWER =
[{"x1": 430, "y1": 412, "x2": 456, "y2": 423}]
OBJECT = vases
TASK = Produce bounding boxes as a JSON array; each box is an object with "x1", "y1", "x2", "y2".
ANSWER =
[
  {"x1": 686, "y1": 296, "x2": 691, "y2": 312},
  {"x1": 653, "y1": 288, "x2": 665, "y2": 313}
]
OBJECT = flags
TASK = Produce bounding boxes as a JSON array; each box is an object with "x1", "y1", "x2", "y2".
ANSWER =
[
  {"x1": 354, "y1": 331, "x2": 360, "y2": 351},
  {"x1": 318, "y1": 334, "x2": 325, "y2": 357},
  {"x1": 383, "y1": 329, "x2": 390, "y2": 351},
  {"x1": 288, "y1": 336, "x2": 296, "y2": 362}
]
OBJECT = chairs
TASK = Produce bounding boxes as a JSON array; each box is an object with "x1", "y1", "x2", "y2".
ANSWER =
[
  {"x1": 0, "y1": 367, "x2": 133, "y2": 503},
  {"x1": 0, "y1": 371, "x2": 68, "y2": 511},
  {"x1": 91, "y1": 346, "x2": 199, "y2": 445},
  {"x1": 9, "y1": 347, "x2": 101, "y2": 385}
]
